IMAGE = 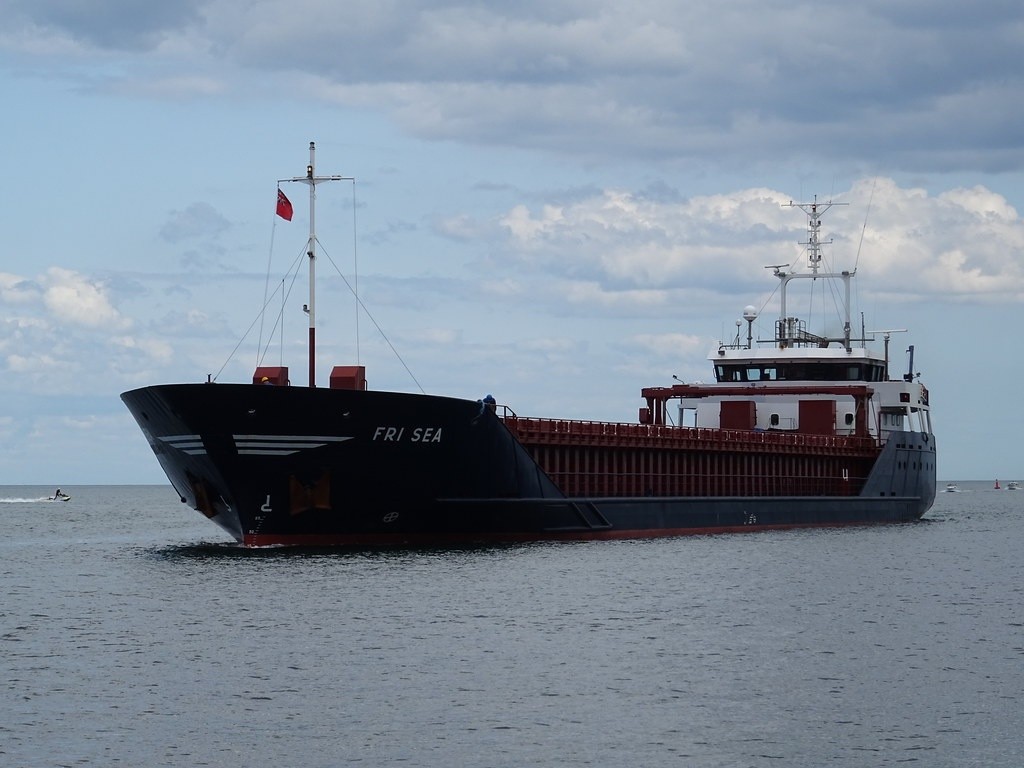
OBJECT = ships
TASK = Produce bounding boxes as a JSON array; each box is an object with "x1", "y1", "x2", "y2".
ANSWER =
[{"x1": 117, "y1": 136, "x2": 939, "y2": 547}]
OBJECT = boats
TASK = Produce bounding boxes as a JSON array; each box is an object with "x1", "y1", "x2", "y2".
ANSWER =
[
  {"x1": 1008, "y1": 481, "x2": 1017, "y2": 489},
  {"x1": 946, "y1": 483, "x2": 957, "y2": 491}
]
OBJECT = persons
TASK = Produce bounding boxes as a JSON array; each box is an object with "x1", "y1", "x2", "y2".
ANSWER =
[
  {"x1": 262, "y1": 377, "x2": 272, "y2": 385},
  {"x1": 54, "y1": 488, "x2": 61, "y2": 500}
]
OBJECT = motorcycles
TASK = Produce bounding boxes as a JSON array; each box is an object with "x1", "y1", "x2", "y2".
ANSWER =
[{"x1": 48, "y1": 492, "x2": 71, "y2": 501}]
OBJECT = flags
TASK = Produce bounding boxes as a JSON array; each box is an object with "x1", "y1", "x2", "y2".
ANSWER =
[{"x1": 276, "y1": 187, "x2": 295, "y2": 223}]
